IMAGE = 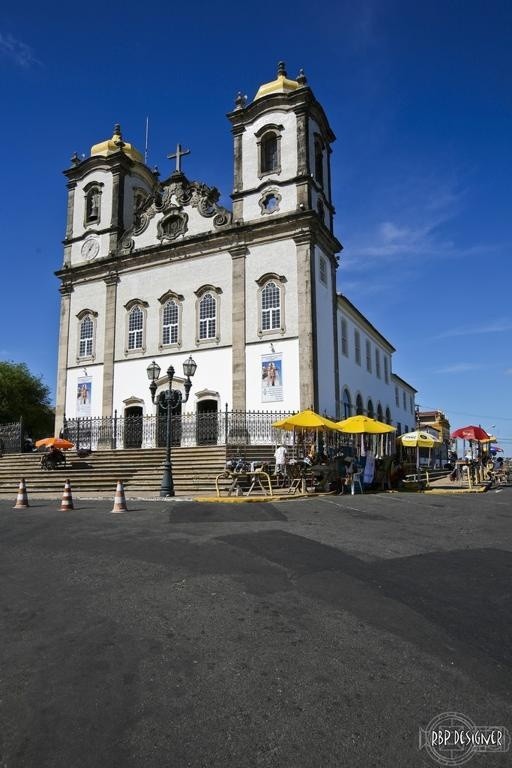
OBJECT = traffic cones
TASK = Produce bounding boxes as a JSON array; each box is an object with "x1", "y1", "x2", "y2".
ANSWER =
[
  {"x1": 108, "y1": 478, "x2": 131, "y2": 514},
  {"x1": 11, "y1": 477, "x2": 33, "y2": 510},
  {"x1": 57, "y1": 477, "x2": 80, "y2": 511}
]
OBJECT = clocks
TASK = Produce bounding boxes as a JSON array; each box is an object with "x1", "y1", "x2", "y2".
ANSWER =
[{"x1": 80, "y1": 237, "x2": 100, "y2": 262}]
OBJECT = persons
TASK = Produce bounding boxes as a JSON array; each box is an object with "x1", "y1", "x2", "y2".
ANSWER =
[
  {"x1": 338, "y1": 459, "x2": 357, "y2": 495},
  {"x1": 273, "y1": 444, "x2": 288, "y2": 487},
  {"x1": 487, "y1": 457, "x2": 503, "y2": 480}
]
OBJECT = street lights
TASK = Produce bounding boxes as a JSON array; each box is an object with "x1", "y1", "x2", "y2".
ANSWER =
[
  {"x1": 144, "y1": 352, "x2": 199, "y2": 497},
  {"x1": 485, "y1": 424, "x2": 497, "y2": 431}
]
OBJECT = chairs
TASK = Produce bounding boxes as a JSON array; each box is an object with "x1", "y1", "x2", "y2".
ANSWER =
[
  {"x1": 340, "y1": 468, "x2": 364, "y2": 495},
  {"x1": 489, "y1": 464, "x2": 508, "y2": 488},
  {"x1": 285, "y1": 461, "x2": 314, "y2": 494}
]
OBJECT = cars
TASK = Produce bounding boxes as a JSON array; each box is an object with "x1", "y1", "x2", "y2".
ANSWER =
[{"x1": 22, "y1": 435, "x2": 38, "y2": 451}]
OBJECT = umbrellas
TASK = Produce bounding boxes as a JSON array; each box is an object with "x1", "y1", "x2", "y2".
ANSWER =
[
  {"x1": 395, "y1": 429, "x2": 440, "y2": 472},
  {"x1": 35, "y1": 437, "x2": 75, "y2": 448},
  {"x1": 335, "y1": 415, "x2": 397, "y2": 457},
  {"x1": 271, "y1": 408, "x2": 343, "y2": 455},
  {"x1": 450, "y1": 425, "x2": 490, "y2": 461}
]
